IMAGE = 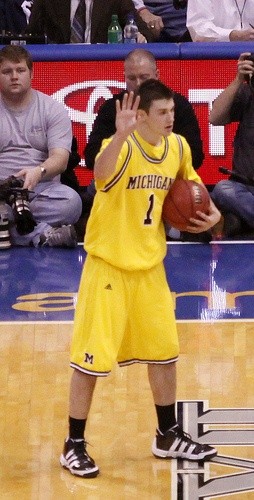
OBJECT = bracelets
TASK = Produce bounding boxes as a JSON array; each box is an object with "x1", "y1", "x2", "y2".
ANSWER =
[{"x1": 136, "y1": 6, "x2": 146, "y2": 12}]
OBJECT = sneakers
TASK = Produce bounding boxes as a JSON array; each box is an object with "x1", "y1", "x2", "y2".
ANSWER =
[
  {"x1": 153, "y1": 424, "x2": 217, "y2": 460},
  {"x1": 43, "y1": 225, "x2": 77, "y2": 248},
  {"x1": 59, "y1": 435, "x2": 99, "y2": 479}
]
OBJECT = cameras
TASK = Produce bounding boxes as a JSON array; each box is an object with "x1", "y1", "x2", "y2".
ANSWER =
[{"x1": 0, "y1": 174, "x2": 34, "y2": 235}]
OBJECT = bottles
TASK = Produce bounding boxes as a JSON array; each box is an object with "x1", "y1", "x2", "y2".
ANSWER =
[
  {"x1": 107, "y1": 15, "x2": 123, "y2": 45},
  {"x1": 123, "y1": 15, "x2": 138, "y2": 45}
]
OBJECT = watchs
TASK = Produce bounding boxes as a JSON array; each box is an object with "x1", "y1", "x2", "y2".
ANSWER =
[{"x1": 40, "y1": 167, "x2": 46, "y2": 174}]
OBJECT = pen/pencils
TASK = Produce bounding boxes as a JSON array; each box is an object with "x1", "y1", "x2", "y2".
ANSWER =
[{"x1": 248, "y1": 22, "x2": 253, "y2": 30}]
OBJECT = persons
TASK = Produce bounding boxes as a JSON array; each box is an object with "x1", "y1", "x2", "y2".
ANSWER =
[
  {"x1": 0, "y1": 45, "x2": 84, "y2": 251},
  {"x1": 59, "y1": 78, "x2": 219, "y2": 479},
  {"x1": 208, "y1": 50, "x2": 254, "y2": 241},
  {"x1": 0, "y1": 0, "x2": 254, "y2": 43},
  {"x1": 83, "y1": 49, "x2": 214, "y2": 243}
]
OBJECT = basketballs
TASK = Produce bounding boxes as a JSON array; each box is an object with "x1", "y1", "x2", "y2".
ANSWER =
[{"x1": 162, "y1": 179, "x2": 210, "y2": 231}]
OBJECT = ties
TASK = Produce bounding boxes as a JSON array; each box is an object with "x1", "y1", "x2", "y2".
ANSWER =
[{"x1": 71, "y1": 0, "x2": 86, "y2": 44}]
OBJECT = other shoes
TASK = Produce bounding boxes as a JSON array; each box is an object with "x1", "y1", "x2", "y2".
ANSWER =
[
  {"x1": 223, "y1": 212, "x2": 246, "y2": 236},
  {"x1": 180, "y1": 230, "x2": 213, "y2": 242}
]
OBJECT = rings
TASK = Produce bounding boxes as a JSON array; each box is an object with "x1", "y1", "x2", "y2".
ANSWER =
[{"x1": 151, "y1": 26, "x2": 154, "y2": 28}]
